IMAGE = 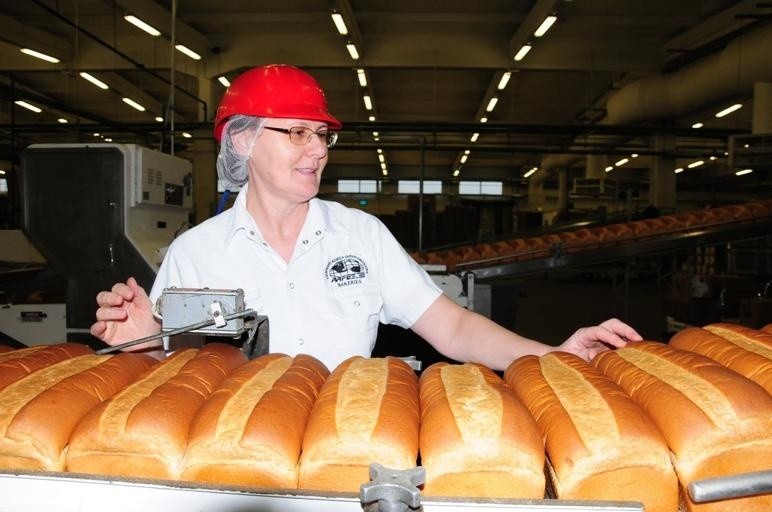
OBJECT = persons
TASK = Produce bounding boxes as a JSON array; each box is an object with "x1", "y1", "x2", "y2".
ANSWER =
[{"x1": 90, "y1": 63, "x2": 645, "y2": 372}]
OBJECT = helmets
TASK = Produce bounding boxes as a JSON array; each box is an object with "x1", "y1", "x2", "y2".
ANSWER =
[{"x1": 214, "y1": 64, "x2": 342, "y2": 142}]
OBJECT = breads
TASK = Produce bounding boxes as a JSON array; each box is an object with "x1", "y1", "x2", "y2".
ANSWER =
[
  {"x1": 0, "y1": 323, "x2": 772, "y2": 512},
  {"x1": 410, "y1": 202, "x2": 771, "y2": 271}
]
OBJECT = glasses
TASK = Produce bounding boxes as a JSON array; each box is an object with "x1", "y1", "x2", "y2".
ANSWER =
[{"x1": 263, "y1": 126, "x2": 338, "y2": 148}]
